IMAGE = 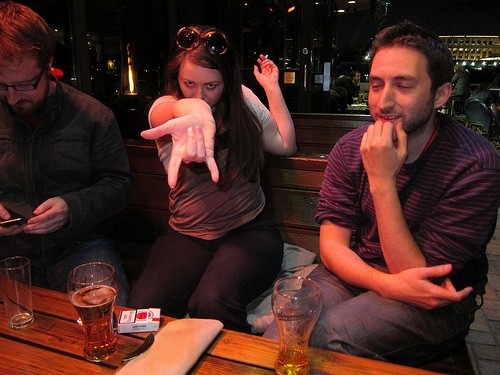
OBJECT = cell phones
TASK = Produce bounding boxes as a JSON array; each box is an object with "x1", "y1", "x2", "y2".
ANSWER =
[{"x1": 0, "y1": 217, "x2": 24, "y2": 226}]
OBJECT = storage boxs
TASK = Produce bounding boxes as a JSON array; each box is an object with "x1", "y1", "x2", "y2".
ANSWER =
[{"x1": 117, "y1": 308, "x2": 162, "y2": 333}]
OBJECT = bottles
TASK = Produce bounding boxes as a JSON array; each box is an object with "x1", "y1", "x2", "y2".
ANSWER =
[{"x1": 120, "y1": 42, "x2": 139, "y2": 98}]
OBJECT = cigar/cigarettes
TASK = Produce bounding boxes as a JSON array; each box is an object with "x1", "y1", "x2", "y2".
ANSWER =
[{"x1": 265, "y1": 54, "x2": 269, "y2": 58}]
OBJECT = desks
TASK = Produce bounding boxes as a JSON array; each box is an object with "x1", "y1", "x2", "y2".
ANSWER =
[{"x1": 0, "y1": 275, "x2": 446, "y2": 375}]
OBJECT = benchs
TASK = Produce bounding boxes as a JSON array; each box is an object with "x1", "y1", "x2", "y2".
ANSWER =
[{"x1": 124, "y1": 113, "x2": 474, "y2": 375}]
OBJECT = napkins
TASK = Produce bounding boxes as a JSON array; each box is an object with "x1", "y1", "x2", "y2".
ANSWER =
[{"x1": 116, "y1": 319, "x2": 224, "y2": 375}]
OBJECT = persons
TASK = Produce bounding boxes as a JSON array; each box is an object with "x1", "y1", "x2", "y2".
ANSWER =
[
  {"x1": 330, "y1": 71, "x2": 360, "y2": 105},
  {"x1": 451, "y1": 66, "x2": 470, "y2": 97},
  {"x1": 263, "y1": 20, "x2": 500, "y2": 357},
  {"x1": 128, "y1": 25, "x2": 297, "y2": 323},
  {"x1": 469, "y1": 83, "x2": 500, "y2": 125},
  {"x1": 0, "y1": 1, "x2": 134, "y2": 307}
]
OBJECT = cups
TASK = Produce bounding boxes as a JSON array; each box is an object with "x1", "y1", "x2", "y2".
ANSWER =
[
  {"x1": 270, "y1": 277, "x2": 323, "y2": 375},
  {"x1": 67, "y1": 262, "x2": 119, "y2": 363},
  {"x1": 0, "y1": 256, "x2": 34, "y2": 328}
]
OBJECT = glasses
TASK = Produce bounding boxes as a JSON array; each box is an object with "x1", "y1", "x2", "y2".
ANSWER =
[
  {"x1": 0, "y1": 60, "x2": 48, "y2": 91},
  {"x1": 175, "y1": 26, "x2": 229, "y2": 55}
]
